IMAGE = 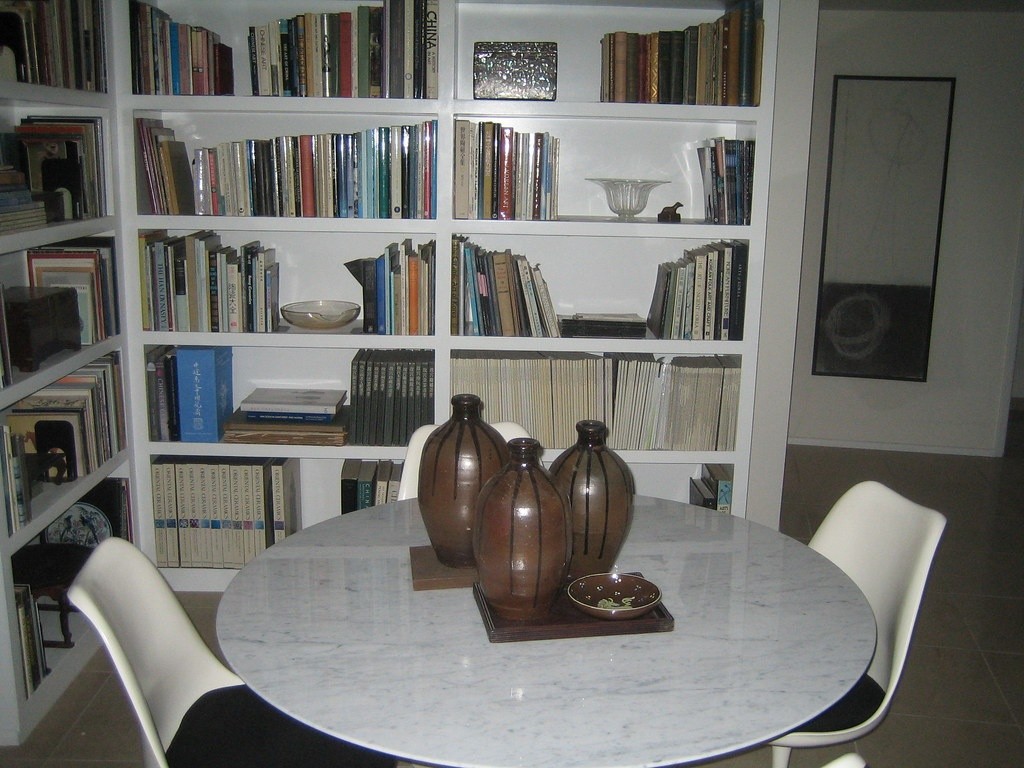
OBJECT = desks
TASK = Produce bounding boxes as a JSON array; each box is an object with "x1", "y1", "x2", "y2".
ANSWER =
[{"x1": 215, "y1": 496, "x2": 877, "y2": 768}]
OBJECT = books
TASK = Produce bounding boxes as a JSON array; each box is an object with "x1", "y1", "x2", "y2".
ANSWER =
[
  {"x1": 129, "y1": 0, "x2": 439, "y2": 99},
  {"x1": 344, "y1": 238, "x2": 435, "y2": 335},
  {"x1": 0, "y1": 0, "x2": 109, "y2": 93},
  {"x1": 138, "y1": 231, "x2": 280, "y2": 332},
  {"x1": 0, "y1": 236, "x2": 121, "y2": 388},
  {"x1": 222, "y1": 387, "x2": 349, "y2": 445},
  {"x1": 340, "y1": 459, "x2": 403, "y2": 515},
  {"x1": 455, "y1": 120, "x2": 560, "y2": 221},
  {"x1": 0, "y1": 352, "x2": 126, "y2": 537},
  {"x1": 39, "y1": 477, "x2": 133, "y2": 551},
  {"x1": 0, "y1": 114, "x2": 108, "y2": 232},
  {"x1": 450, "y1": 349, "x2": 742, "y2": 452},
  {"x1": 689, "y1": 463, "x2": 732, "y2": 514},
  {"x1": 349, "y1": 349, "x2": 435, "y2": 446},
  {"x1": 146, "y1": 345, "x2": 233, "y2": 442},
  {"x1": 13, "y1": 584, "x2": 47, "y2": 699},
  {"x1": 450, "y1": 236, "x2": 748, "y2": 340},
  {"x1": 136, "y1": 116, "x2": 436, "y2": 219},
  {"x1": 151, "y1": 457, "x2": 301, "y2": 569},
  {"x1": 598, "y1": 0, "x2": 765, "y2": 106},
  {"x1": 696, "y1": 136, "x2": 755, "y2": 226}
]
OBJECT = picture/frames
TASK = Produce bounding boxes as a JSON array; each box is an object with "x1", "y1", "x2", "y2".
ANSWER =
[{"x1": 808, "y1": 75, "x2": 957, "y2": 383}]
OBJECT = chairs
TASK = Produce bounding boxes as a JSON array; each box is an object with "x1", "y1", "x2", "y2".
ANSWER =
[
  {"x1": 396, "y1": 421, "x2": 544, "y2": 501},
  {"x1": 67, "y1": 536, "x2": 397, "y2": 768},
  {"x1": 761, "y1": 480, "x2": 947, "y2": 768}
]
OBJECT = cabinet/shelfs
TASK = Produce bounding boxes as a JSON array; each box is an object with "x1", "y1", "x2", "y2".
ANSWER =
[{"x1": 0, "y1": 0, "x2": 782, "y2": 745}]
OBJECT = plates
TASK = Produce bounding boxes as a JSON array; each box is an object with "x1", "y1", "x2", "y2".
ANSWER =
[
  {"x1": 567, "y1": 572, "x2": 662, "y2": 621},
  {"x1": 280, "y1": 299, "x2": 361, "y2": 328}
]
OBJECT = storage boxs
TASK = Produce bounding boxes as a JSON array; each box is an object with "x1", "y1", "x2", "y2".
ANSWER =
[
  {"x1": 473, "y1": 41, "x2": 558, "y2": 102},
  {"x1": 3, "y1": 286, "x2": 82, "y2": 373}
]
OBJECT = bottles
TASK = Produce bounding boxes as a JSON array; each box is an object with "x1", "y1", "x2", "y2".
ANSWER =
[
  {"x1": 472, "y1": 438, "x2": 574, "y2": 622},
  {"x1": 548, "y1": 420, "x2": 635, "y2": 581},
  {"x1": 418, "y1": 393, "x2": 508, "y2": 569}
]
